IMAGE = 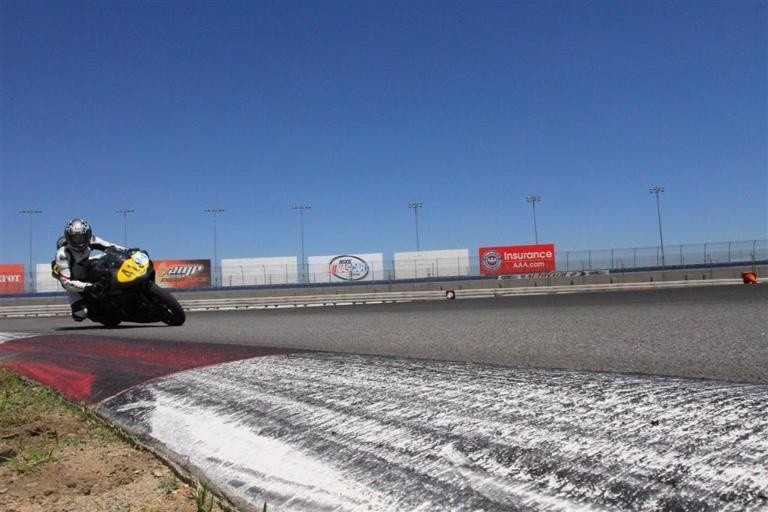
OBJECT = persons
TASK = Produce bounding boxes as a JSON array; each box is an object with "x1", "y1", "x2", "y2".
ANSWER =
[{"x1": 49, "y1": 217, "x2": 129, "y2": 322}]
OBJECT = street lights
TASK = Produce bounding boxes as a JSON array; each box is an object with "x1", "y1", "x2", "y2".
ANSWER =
[
  {"x1": 290, "y1": 200, "x2": 312, "y2": 282},
  {"x1": 526, "y1": 192, "x2": 541, "y2": 244},
  {"x1": 15, "y1": 208, "x2": 43, "y2": 293},
  {"x1": 408, "y1": 199, "x2": 424, "y2": 249},
  {"x1": 646, "y1": 182, "x2": 665, "y2": 268},
  {"x1": 203, "y1": 207, "x2": 223, "y2": 287},
  {"x1": 113, "y1": 208, "x2": 136, "y2": 249}
]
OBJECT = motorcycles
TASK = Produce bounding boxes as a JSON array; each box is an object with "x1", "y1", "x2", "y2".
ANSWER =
[{"x1": 75, "y1": 244, "x2": 188, "y2": 328}]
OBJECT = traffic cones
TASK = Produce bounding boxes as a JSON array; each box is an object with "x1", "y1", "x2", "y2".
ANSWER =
[
  {"x1": 740, "y1": 271, "x2": 756, "y2": 284},
  {"x1": 444, "y1": 289, "x2": 455, "y2": 300}
]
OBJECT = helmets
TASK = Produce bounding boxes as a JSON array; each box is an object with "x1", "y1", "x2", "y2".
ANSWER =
[
  {"x1": 64, "y1": 218, "x2": 91, "y2": 252},
  {"x1": 56, "y1": 235, "x2": 69, "y2": 251}
]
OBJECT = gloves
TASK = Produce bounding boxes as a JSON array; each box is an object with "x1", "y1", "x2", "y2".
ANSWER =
[{"x1": 85, "y1": 282, "x2": 106, "y2": 301}]
